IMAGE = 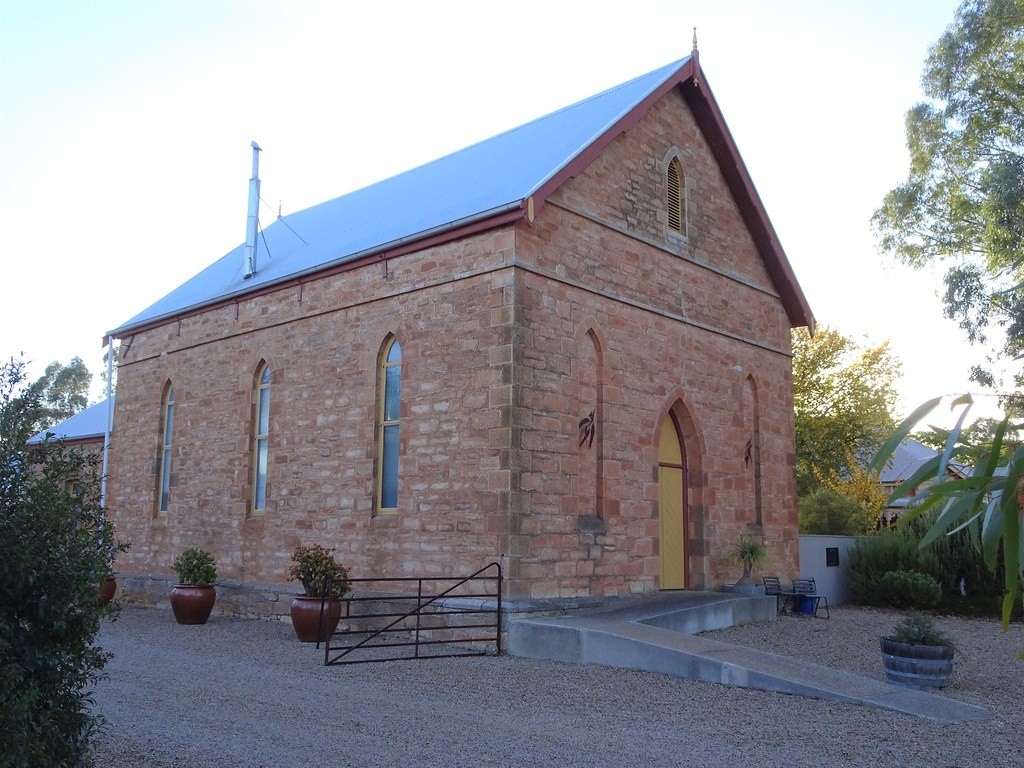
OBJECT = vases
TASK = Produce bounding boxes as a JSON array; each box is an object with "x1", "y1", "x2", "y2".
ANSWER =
[{"x1": 94, "y1": 577, "x2": 116, "y2": 609}]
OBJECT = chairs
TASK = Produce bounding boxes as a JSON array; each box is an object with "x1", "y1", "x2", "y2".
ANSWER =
[
  {"x1": 790, "y1": 577, "x2": 830, "y2": 620},
  {"x1": 762, "y1": 576, "x2": 800, "y2": 617}
]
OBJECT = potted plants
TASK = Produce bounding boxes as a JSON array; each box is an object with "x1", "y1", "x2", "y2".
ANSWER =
[
  {"x1": 880, "y1": 611, "x2": 966, "y2": 692},
  {"x1": 285, "y1": 543, "x2": 352, "y2": 643},
  {"x1": 167, "y1": 545, "x2": 219, "y2": 625}
]
